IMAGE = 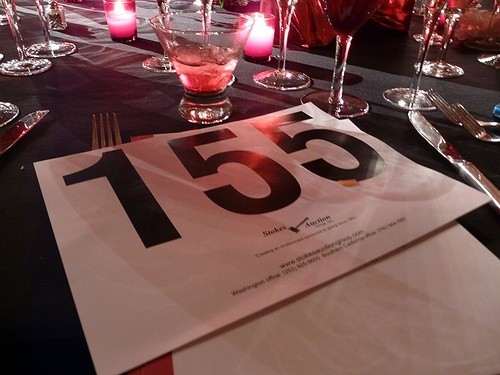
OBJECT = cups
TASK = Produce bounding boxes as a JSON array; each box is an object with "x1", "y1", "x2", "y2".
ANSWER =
[
  {"x1": 242, "y1": 12, "x2": 275, "y2": 62},
  {"x1": 104, "y1": 0, "x2": 138, "y2": 44},
  {"x1": 150, "y1": 11, "x2": 247, "y2": 124}
]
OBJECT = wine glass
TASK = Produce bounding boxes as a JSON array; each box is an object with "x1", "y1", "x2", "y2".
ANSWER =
[
  {"x1": 142, "y1": 0, "x2": 179, "y2": 72},
  {"x1": 251, "y1": 0, "x2": 310, "y2": 91},
  {"x1": 301, "y1": 0, "x2": 383, "y2": 119},
  {"x1": 384, "y1": 0, "x2": 446, "y2": 111},
  {"x1": 0, "y1": 0, "x2": 52, "y2": 76},
  {"x1": 24, "y1": 0, "x2": 77, "y2": 58},
  {"x1": 413, "y1": 0, "x2": 453, "y2": 45},
  {"x1": 415, "y1": 0, "x2": 464, "y2": 76},
  {"x1": 201, "y1": 0, "x2": 214, "y2": 56}
]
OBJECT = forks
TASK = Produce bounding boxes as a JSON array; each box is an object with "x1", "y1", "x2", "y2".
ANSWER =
[
  {"x1": 91, "y1": 113, "x2": 121, "y2": 151},
  {"x1": 425, "y1": 88, "x2": 500, "y2": 128},
  {"x1": 450, "y1": 103, "x2": 500, "y2": 146}
]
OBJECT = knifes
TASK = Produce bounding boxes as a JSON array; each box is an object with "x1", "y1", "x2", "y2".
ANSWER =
[
  {"x1": 408, "y1": 111, "x2": 500, "y2": 213},
  {"x1": 0, "y1": 110, "x2": 54, "y2": 155}
]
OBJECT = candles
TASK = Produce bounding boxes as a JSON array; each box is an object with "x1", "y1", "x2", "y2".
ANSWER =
[
  {"x1": 103, "y1": 0, "x2": 137, "y2": 43},
  {"x1": 240, "y1": 13, "x2": 276, "y2": 62}
]
